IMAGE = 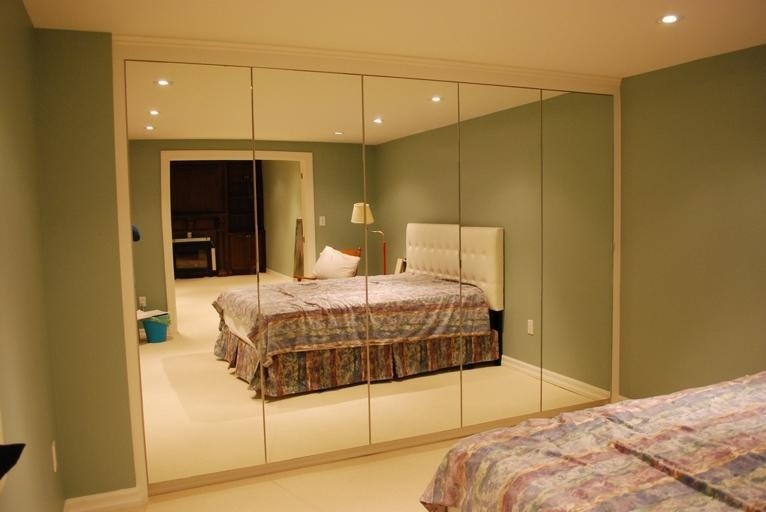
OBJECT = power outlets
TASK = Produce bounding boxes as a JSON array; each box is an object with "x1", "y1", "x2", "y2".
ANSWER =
[
  {"x1": 527, "y1": 319, "x2": 534, "y2": 335},
  {"x1": 138, "y1": 297, "x2": 146, "y2": 310}
]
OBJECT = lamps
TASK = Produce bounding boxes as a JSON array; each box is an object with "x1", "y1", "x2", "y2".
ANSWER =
[{"x1": 351, "y1": 202, "x2": 386, "y2": 276}]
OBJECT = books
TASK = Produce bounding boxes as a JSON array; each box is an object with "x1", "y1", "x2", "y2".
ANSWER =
[{"x1": 391, "y1": 257, "x2": 406, "y2": 275}]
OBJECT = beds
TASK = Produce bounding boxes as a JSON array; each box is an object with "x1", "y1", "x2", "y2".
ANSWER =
[
  {"x1": 416, "y1": 370, "x2": 766, "y2": 512},
  {"x1": 214, "y1": 222, "x2": 507, "y2": 400}
]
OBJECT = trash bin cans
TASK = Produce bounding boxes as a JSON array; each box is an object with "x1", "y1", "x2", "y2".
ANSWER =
[{"x1": 140, "y1": 313, "x2": 169, "y2": 343}]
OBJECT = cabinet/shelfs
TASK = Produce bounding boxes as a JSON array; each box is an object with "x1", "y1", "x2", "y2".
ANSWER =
[{"x1": 173, "y1": 211, "x2": 260, "y2": 276}]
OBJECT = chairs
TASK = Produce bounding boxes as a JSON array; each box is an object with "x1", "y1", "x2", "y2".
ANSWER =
[{"x1": 341, "y1": 248, "x2": 360, "y2": 256}]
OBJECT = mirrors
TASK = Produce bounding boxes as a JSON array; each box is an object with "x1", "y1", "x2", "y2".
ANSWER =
[
  {"x1": 251, "y1": 62, "x2": 370, "y2": 470},
  {"x1": 541, "y1": 61, "x2": 618, "y2": 419},
  {"x1": 371, "y1": 60, "x2": 464, "y2": 454},
  {"x1": 124, "y1": 60, "x2": 267, "y2": 494},
  {"x1": 464, "y1": 60, "x2": 541, "y2": 439}
]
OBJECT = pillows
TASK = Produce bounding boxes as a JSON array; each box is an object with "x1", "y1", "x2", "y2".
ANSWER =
[{"x1": 315, "y1": 245, "x2": 361, "y2": 280}]
